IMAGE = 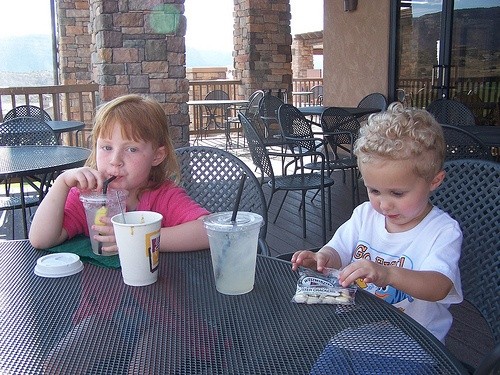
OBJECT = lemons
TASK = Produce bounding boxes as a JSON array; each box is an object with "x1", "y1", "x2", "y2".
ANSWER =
[{"x1": 95, "y1": 205, "x2": 107, "y2": 234}]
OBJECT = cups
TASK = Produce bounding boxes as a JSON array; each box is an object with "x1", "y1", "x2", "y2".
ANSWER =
[
  {"x1": 202, "y1": 211, "x2": 265, "y2": 296},
  {"x1": 110, "y1": 211, "x2": 164, "y2": 287},
  {"x1": 79, "y1": 187, "x2": 130, "y2": 256}
]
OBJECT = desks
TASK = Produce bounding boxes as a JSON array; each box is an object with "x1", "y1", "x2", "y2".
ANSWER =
[
  {"x1": 0, "y1": 145, "x2": 93, "y2": 239},
  {"x1": 185, "y1": 98, "x2": 250, "y2": 149},
  {"x1": 0, "y1": 120, "x2": 85, "y2": 145},
  {"x1": 275, "y1": 105, "x2": 382, "y2": 182},
  {"x1": 0, "y1": 239, "x2": 471, "y2": 375},
  {"x1": 442, "y1": 126, "x2": 499, "y2": 152}
]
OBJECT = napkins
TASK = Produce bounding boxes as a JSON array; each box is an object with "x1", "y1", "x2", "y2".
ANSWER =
[{"x1": 45, "y1": 232, "x2": 122, "y2": 271}]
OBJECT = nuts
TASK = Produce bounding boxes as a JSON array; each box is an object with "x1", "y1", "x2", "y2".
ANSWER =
[{"x1": 295, "y1": 287, "x2": 352, "y2": 304}]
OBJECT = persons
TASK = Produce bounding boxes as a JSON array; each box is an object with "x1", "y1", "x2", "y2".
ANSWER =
[
  {"x1": 291, "y1": 102, "x2": 463, "y2": 375},
  {"x1": 28, "y1": 95, "x2": 237, "y2": 375}
]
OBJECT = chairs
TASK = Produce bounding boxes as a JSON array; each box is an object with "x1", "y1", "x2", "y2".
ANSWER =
[{"x1": 0, "y1": 85, "x2": 500, "y2": 375}]
